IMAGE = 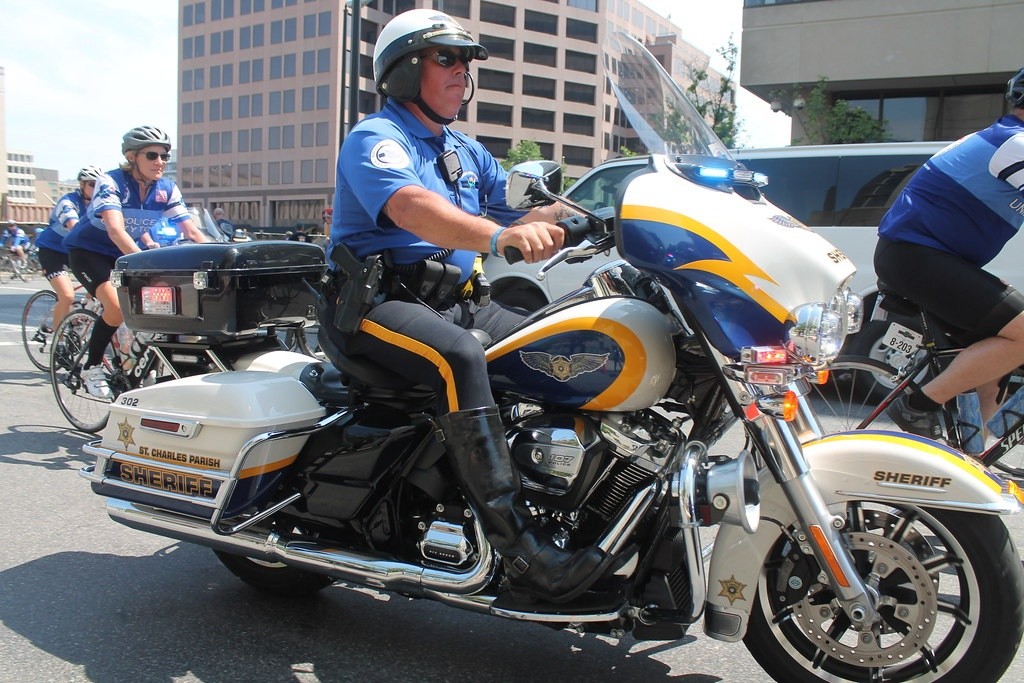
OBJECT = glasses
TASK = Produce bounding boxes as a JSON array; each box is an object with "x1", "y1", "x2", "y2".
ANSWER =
[
  {"x1": 83, "y1": 180, "x2": 96, "y2": 188},
  {"x1": 417, "y1": 49, "x2": 471, "y2": 72},
  {"x1": 137, "y1": 150, "x2": 172, "y2": 162}
]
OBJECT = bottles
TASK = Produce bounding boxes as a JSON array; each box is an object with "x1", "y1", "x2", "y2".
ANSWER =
[
  {"x1": 89, "y1": 300, "x2": 103, "y2": 320},
  {"x1": 986, "y1": 385, "x2": 1024, "y2": 438},
  {"x1": 116, "y1": 322, "x2": 133, "y2": 370},
  {"x1": 129, "y1": 332, "x2": 154, "y2": 358},
  {"x1": 956, "y1": 387, "x2": 984, "y2": 454}
]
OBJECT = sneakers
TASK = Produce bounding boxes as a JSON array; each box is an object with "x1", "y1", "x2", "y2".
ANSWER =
[
  {"x1": 79, "y1": 363, "x2": 113, "y2": 398},
  {"x1": 885, "y1": 393, "x2": 943, "y2": 440}
]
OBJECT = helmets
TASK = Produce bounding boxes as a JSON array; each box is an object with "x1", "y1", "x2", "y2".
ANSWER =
[
  {"x1": 35, "y1": 228, "x2": 46, "y2": 233},
  {"x1": 1006, "y1": 68, "x2": 1024, "y2": 101},
  {"x1": 6, "y1": 219, "x2": 16, "y2": 226},
  {"x1": 78, "y1": 165, "x2": 104, "y2": 182},
  {"x1": 373, "y1": 7, "x2": 490, "y2": 102},
  {"x1": 122, "y1": 126, "x2": 171, "y2": 156}
]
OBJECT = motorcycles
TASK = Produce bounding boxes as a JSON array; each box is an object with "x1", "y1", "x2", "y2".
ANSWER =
[{"x1": 80, "y1": 24, "x2": 1024, "y2": 683}]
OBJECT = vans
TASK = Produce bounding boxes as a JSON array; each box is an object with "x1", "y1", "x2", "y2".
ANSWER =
[{"x1": 544, "y1": 137, "x2": 1024, "y2": 406}]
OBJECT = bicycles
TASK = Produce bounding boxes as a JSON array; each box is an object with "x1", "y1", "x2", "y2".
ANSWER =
[
  {"x1": 47, "y1": 307, "x2": 169, "y2": 434},
  {"x1": 0, "y1": 243, "x2": 44, "y2": 284},
  {"x1": 22, "y1": 278, "x2": 103, "y2": 374},
  {"x1": 785, "y1": 277, "x2": 1024, "y2": 481}
]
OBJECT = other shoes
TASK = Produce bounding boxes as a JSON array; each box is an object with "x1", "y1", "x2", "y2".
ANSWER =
[
  {"x1": 53, "y1": 345, "x2": 70, "y2": 364},
  {"x1": 21, "y1": 260, "x2": 28, "y2": 268}
]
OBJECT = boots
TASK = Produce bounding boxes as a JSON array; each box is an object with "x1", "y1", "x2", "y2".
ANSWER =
[{"x1": 437, "y1": 404, "x2": 614, "y2": 604}]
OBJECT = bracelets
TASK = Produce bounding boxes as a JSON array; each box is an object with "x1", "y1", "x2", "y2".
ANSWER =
[{"x1": 490, "y1": 227, "x2": 507, "y2": 259}]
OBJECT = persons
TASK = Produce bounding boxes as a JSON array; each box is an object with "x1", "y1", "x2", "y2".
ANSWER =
[
  {"x1": 214, "y1": 208, "x2": 234, "y2": 240},
  {"x1": 289, "y1": 223, "x2": 312, "y2": 243},
  {"x1": 874, "y1": 66, "x2": 1024, "y2": 447},
  {"x1": 62, "y1": 125, "x2": 211, "y2": 400},
  {"x1": 136, "y1": 218, "x2": 181, "y2": 251},
  {"x1": 286, "y1": 231, "x2": 293, "y2": 240},
  {"x1": 35, "y1": 164, "x2": 105, "y2": 338},
  {"x1": 0, "y1": 220, "x2": 31, "y2": 279},
  {"x1": 315, "y1": 8, "x2": 613, "y2": 602},
  {"x1": 30, "y1": 228, "x2": 44, "y2": 252}
]
